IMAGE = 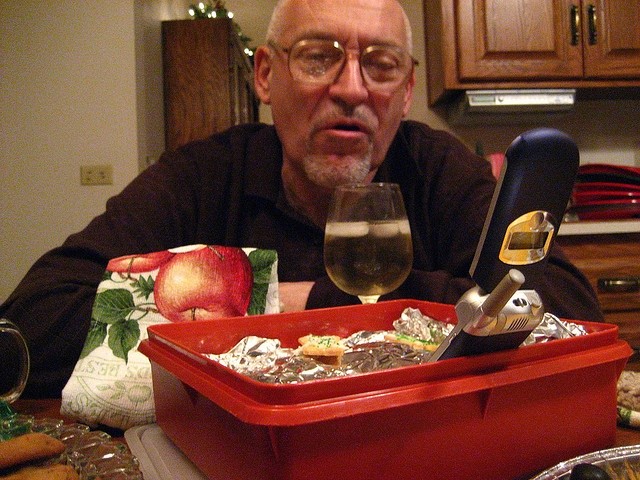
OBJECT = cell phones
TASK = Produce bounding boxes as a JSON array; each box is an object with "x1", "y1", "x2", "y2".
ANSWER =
[{"x1": 428, "y1": 128, "x2": 580, "y2": 363}]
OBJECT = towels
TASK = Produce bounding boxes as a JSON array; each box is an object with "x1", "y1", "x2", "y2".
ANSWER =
[{"x1": 59, "y1": 243, "x2": 285, "y2": 431}]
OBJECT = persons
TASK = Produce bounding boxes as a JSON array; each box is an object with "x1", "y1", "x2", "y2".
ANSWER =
[{"x1": 0, "y1": 0, "x2": 603, "y2": 401}]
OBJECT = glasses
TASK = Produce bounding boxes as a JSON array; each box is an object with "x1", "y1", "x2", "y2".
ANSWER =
[{"x1": 265, "y1": 38, "x2": 420, "y2": 100}]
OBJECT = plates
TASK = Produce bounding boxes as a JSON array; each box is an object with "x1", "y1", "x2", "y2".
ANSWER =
[
  {"x1": 1, "y1": 414, "x2": 144, "y2": 480},
  {"x1": 569, "y1": 161, "x2": 640, "y2": 222}
]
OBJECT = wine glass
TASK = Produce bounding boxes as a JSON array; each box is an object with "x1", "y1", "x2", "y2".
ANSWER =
[{"x1": 323, "y1": 182, "x2": 415, "y2": 306}]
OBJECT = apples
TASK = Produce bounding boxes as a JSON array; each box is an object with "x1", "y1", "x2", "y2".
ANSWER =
[
  {"x1": 107, "y1": 251, "x2": 174, "y2": 272},
  {"x1": 154, "y1": 246, "x2": 252, "y2": 323}
]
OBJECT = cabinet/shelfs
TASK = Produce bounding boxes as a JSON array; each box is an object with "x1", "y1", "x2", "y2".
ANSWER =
[
  {"x1": 161, "y1": 18, "x2": 260, "y2": 148},
  {"x1": 552, "y1": 220, "x2": 640, "y2": 373},
  {"x1": 423, "y1": 0, "x2": 639, "y2": 106}
]
